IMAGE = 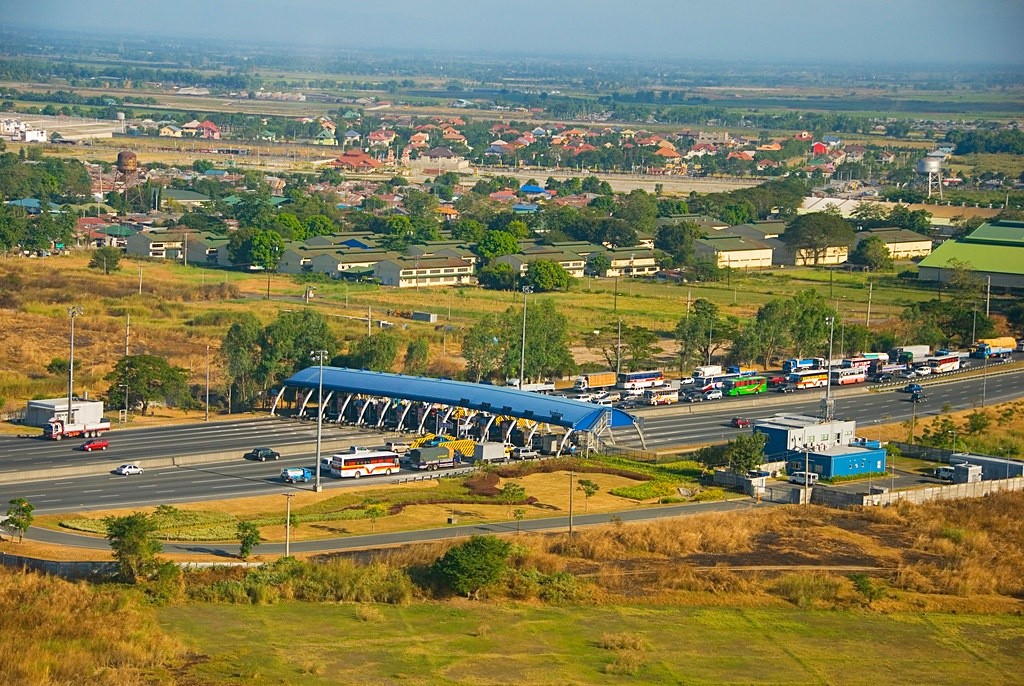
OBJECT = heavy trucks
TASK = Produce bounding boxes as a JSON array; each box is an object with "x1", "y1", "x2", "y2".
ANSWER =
[{"x1": 43, "y1": 416, "x2": 111, "y2": 441}]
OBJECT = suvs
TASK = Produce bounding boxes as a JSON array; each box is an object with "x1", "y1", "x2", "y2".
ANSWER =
[
  {"x1": 731, "y1": 416, "x2": 751, "y2": 429},
  {"x1": 251, "y1": 447, "x2": 281, "y2": 462},
  {"x1": 80, "y1": 438, "x2": 109, "y2": 452}
]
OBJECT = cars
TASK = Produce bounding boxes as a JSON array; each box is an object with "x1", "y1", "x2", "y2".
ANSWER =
[
  {"x1": 321, "y1": 436, "x2": 540, "y2": 472},
  {"x1": 116, "y1": 464, "x2": 144, "y2": 476}
]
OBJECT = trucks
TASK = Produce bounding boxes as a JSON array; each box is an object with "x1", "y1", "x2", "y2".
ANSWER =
[{"x1": 279, "y1": 467, "x2": 312, "y2": 484}]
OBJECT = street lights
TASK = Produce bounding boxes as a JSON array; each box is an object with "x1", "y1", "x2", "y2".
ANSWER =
[
  {"x1": 66, "y1": 306, "x2": 84, "y2": 424},
  {"x1": 873, "y1": 419, "x2": 884, "y2": 440},
  {"x1": 824, "y1": 315, "x2": 835, "y2": 419},
  {"x1": 998, "y1": 447, "x2": 1010, "y2": 479},
  {"x1": 518, "y1": 283, "x2": 535, "y2": 390},
  {"x1": 309, "y1": 349, "x2": 330, "y2": 492},
  {"x1": 948, "y1": 430, "x2": 956, "y2": 453}
]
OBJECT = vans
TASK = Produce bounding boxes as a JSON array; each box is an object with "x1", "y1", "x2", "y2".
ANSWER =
[
  {"x1": 934, "y1": 465, "x2": 955, "y2": 480},
  {"x1": 790, "y1": 472, "x2": 819, "y2": 486}
]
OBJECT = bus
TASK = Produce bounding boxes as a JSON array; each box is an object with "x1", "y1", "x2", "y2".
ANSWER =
[
  {"x1": 506, "y1": 334, "x2": 1024, "y2": 408},
  {"x1": 331, "y1": 451, "x2": 401, "y2": 479}
]
